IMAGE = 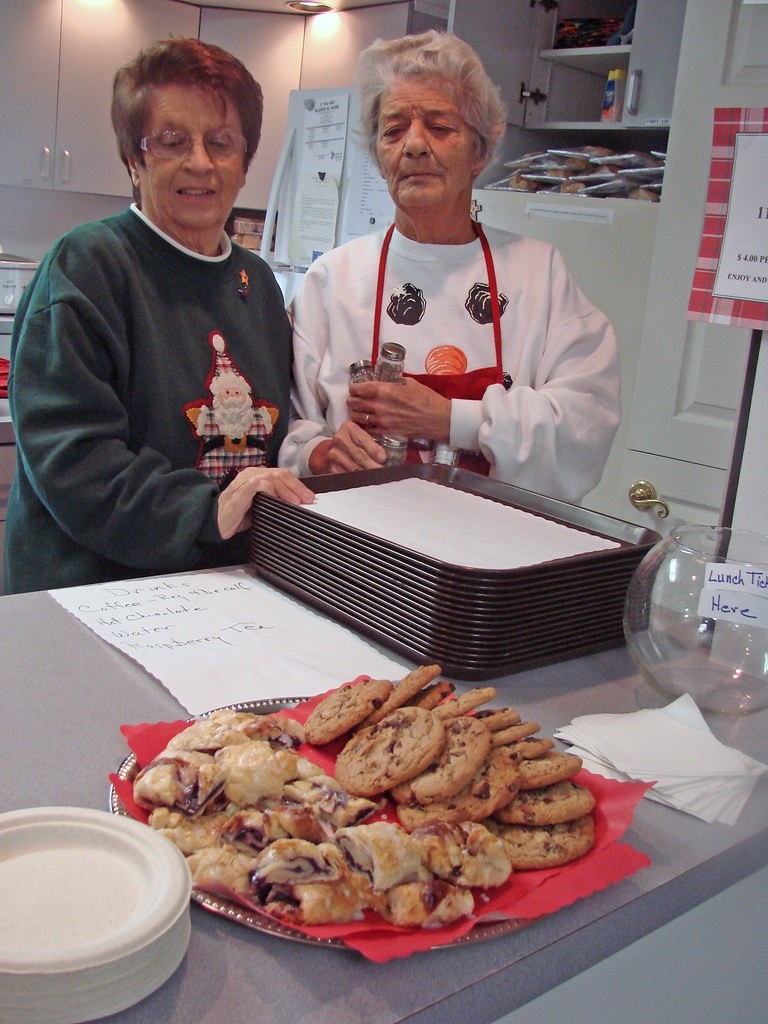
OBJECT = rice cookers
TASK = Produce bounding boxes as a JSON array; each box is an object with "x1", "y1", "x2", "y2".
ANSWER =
[{"x1": 0, "y1": 244, "x2": 41, "y2": 314}]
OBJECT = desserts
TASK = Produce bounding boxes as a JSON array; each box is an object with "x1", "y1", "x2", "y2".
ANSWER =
[{"x1": 130, "y1": 708, "x2": 515, "y2": 929}]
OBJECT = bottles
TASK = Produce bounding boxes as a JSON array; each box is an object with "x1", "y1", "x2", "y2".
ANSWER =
[
  {"x1": 379, "y1": 435, "x2": 408, "y2": 468},
  {"x1": 347, "y1": 359, "x2": 375, "y2": 389},
  {"x1": 600, "y1": 67, "x2": 626, "y2": 122},
  {"x1": 373, "y1": 343, "x2": 407, "y2": 383}
]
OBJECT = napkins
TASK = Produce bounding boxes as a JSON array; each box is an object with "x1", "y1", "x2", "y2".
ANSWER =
[{"x1": 554, "y1": 689, "x2": 768, "y2": 827}]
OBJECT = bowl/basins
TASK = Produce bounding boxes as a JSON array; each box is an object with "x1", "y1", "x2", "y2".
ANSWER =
[
  {"x1": 0, "y1": 399, "x2": 17, "y2": 444},
  {"x1": 233, "y1": 221, "x2": 276, "y2": 251},
  {"x1": 622, "y1": 524, "x2": 768, "y2": 715}
]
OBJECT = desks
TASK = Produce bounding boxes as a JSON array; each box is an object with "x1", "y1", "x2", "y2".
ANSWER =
[{"x1": 0, "y1": 565, "x2": 768, "y2": 1024}]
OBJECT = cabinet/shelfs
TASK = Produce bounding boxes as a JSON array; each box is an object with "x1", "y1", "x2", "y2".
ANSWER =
[{"x1": 0, "y1": 0, "x2": 686, "y2": 220}]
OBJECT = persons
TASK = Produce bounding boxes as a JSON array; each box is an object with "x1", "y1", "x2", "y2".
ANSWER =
[
  {"x1": 279, "y1": 29, "x2": 622, "y2": 507},
  {"x1": 3, "y1": 36, "x2": 316, "y2": 599}
]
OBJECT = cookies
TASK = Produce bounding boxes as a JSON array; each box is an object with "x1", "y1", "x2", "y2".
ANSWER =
[{"x1": 302, "y1": 660, "x2": 595, "y2": 870}]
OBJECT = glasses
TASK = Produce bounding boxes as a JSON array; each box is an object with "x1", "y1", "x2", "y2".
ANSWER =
[{"x1": 137, "y1": 129, "x2": 247, "y2": 160}]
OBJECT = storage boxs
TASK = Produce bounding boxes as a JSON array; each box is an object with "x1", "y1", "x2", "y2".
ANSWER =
[{"x1": 229, "y1": 221, "x2": 278, "y2": 250}]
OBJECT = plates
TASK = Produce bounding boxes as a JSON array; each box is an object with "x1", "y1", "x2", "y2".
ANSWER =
[
  {"x1": 0, "y1": 804, "x2": 193, "y2": 1024},
  {"x1": 109, "y1": 695, "x2": 544, "y2": 950}
]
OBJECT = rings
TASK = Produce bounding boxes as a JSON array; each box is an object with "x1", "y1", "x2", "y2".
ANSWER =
[{"x1": 363, "y1": 413, "x2": 372, "y2": 422}]
